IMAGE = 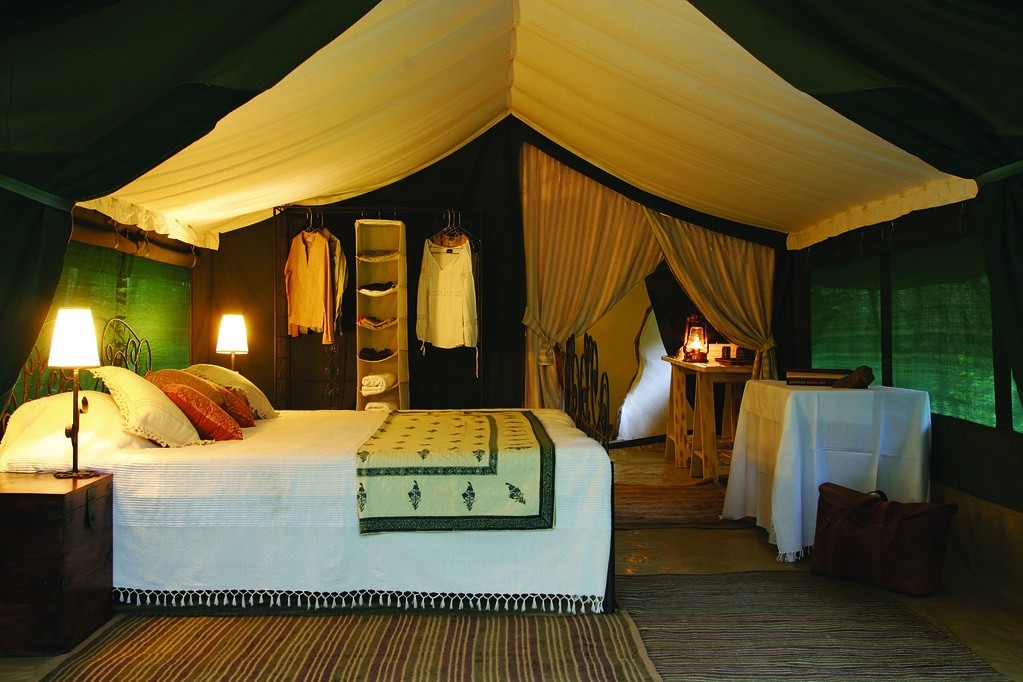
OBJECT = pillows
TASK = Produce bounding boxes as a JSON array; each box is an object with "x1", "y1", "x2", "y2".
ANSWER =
[
  {"x1": 141, "y1": 368, "x2": 224, "y2": 410},
  {"x1": 82, "y1": 365, "x2": 215, "y2": 450},
  {"x1": 161, "y1": 382, "x2": 244, "y2": 440},
  {"x1": 210, "y1": 383, "x2": 256, "y2": 428},
  {"x1": 180, "y1": 364, "x2": 280, "y2": 420}
]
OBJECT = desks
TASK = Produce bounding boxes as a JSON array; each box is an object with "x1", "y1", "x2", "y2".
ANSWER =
[{"x1": 664, "y1": 354, "x2": 756, "y2": 481}]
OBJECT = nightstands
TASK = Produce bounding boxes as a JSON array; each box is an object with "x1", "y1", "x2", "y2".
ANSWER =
[{"x1": 0, "y1": 468, "x2": 115, "y2": 656}]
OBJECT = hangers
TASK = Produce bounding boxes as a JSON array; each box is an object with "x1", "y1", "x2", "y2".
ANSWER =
[{"x1": 300, "y1": 205, "x2": 485, "y2": 249}]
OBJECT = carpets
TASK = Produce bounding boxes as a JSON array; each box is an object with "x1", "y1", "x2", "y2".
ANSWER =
[
  {"x1": 609, "y1": 480, "x2": 760, "y2": 527},
  {"x1": 612, "y1": 569, "x2": 1020, "y2": 682},
  {"x1": 38, "y1": 605, "x2": 666, "y2": 682}
]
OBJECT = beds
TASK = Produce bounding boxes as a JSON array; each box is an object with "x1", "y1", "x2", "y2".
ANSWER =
[{"x1": 2, "y1": 389, "x2": 620, "y2": 616}]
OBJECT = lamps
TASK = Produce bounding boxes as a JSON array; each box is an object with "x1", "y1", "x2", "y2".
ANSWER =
[
  {"x1": 50, "y1": 306, "x2": 105, "y2": 479},
  {"x1": 214, "y1": 311, "x2": 249, "y2": 372}
]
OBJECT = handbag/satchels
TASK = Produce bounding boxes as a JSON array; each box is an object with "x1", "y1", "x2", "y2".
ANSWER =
[{"x1": 809, "y1": 483, "x2": 957, "y2": 598}]
